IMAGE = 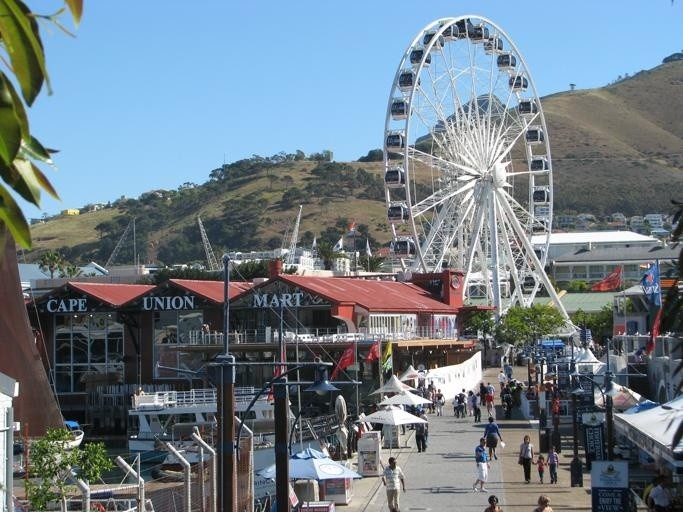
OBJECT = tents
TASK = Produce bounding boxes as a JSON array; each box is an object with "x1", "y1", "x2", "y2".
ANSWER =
[
  {"x1": 562, "y1": 346, "x2": 607, "y2": 374},
  {"x1": 613, "y1": 395, "x2": 683, "y2": 502}
]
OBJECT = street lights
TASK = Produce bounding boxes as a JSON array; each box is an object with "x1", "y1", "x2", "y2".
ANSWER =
[
  {"x1": 569, "y1": 373, "x2": 613, "y2": 461},
  {"x1": 524, "y1": 343, "x2": 649, "y2": 487}
]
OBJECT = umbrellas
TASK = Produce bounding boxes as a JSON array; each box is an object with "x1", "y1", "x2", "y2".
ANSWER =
[
  {"x1": 354, "y1": 405, "x2": 430, "y2": 457},
  {"x1": 376, "y1": 390, "x2": 433, "y2": 406},
  {"x1": 593, "y1": 381, "x2": 660, "y2": 414},
  {"x1": 254, "y1": 443, "x2": 362, "y2": 512},
  {"x1": 368, "y1": 374, "x2": 418, "y2": 396},
  {"x1": 399, "y1": 365, "x2": 425, "y2": 382}
]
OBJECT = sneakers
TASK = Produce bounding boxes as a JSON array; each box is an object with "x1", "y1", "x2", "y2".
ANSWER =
[
  {"x1": 473, "y1": 483, "x2": 477, "y2": 492},
  {"x1": 480, "y1": 488, "x2": 488, "y2": 492}
]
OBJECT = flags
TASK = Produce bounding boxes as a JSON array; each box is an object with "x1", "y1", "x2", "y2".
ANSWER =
[
  {"x1": 382, "y1": 341, "x2": 392, "y2": 373},
  {"x1": 366, "y1": 340, "x2": 379, "y2": 367},
  {"x1": 646, "y1": 309, "x2": 662, "y2": 359},
  {"x1": 333, "y1": 238, "x2": 343, "y2": 251},
  {"x1": 591, "y1": 264, "x2": 622, "y2": 291},
  {"x1": 331, "y1": 341, "x2": 355, "y2": 381},
  {"x1": 640, "y1": 259, "x2": 661, "y2": 305}
]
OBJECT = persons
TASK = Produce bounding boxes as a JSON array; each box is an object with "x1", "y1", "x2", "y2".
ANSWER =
[
  {"x1": 552, "y1": 411, "x2": 560, "y2": 432},
  {"x1": 414, "y1": 414, "x2": 426, "y2": 453},
  {"x1": 502, "y1": 388, "x2": 513, "y2": 420},
  {"x1": 409, "y1": 404, "x2": 419, "y2": 430},
  {"x1": 519, "y1": 435, "x2": 534, "y2": 483},
  {"x1": 467, "y1": 391, "x2": 473, "y2": 416},
  {"x1": 451, "y1": 395, "x2": 458, "y2": 416},
  {"x1": 504, "y1": 364, "x2": 512, "y2": 379},
  {"x1": 483, "y1": 416, "x2": 503, "y2": 461},
  {"x1": 648, "y1": 475, "x2": 673, "y2": 512},
  {"x1": 643, "y1": 477, "x2": 659, "y2": 504},
  {"x1": 473, "y1": 438, "x2": 490, "y2": 492},
  {"x1": 497, "y1": 370, "x2": 506, "y2": 390},
  {"x1": 533, "y1": 455, "x2": 546, "y2": 484},
  {"x1": 482, "y1": 391, "x2": 494, "y2": 416},
  {"x1": 546, "y1": 446, "x2": 559, "y2": 484},
  {"x1": 420, "y1": 410, "x2": 428, "y2": 440},
  {"x1": 431, "y1": 381, "x2": 436, "y2": 405},
  {"x1": 427, "y1": 387, "x2": 432, "y2": 414},
  {"x1": 634, "y1": 347, "x2": 646, "y2": 363},
  {"x1": 457, "y1": 393, "x2": 465, "y2": 419},
  {"x1": 434, "y1": 389, "x2": 445, "y2": 416},
  {"x1": 397, "y1": 404, "x2": 406, "y2": 435},
  {"x1": 381, "y1": 457, "x2": 405, "y2": 512},
  {"x1": 472, "y1": 391, "x2": 481, "y2": 423},
  {"x1": 532, "y1": 496, "x2": 553, "y2": 512},
  {"x1": 500, "y1": 382, "x2": 507, "y2": 414},
  {"x1": 417, "y1": 382, "x2": 424, "y2": 398},
  {"x1": 539, "y1": 409, "x2": 547, "y2": 431},
  {"x1": 462, "y1": 389, "x2": 468, "y2": 415},
  {"x1": 484, "y1": 495, "x2": 503, "y2": 512}
]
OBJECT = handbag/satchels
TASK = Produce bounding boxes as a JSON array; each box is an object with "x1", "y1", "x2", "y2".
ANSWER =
[{"x1": 518, "y1": 444, "x2": 529, "y2": 465}]
